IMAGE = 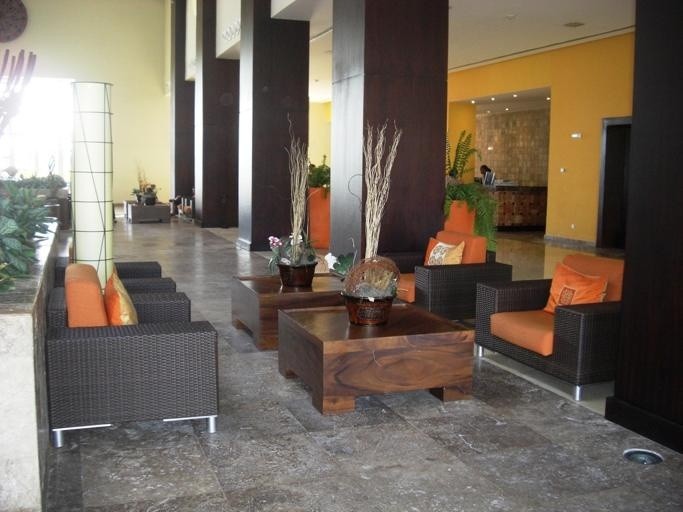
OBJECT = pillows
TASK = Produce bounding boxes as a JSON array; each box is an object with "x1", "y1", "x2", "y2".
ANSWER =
[
  {"x1": 543, "y1": 259, "x2": 610, "y2": 315},
  {"x1": 423, "y1": 237, "x2": 466, "y2": 267},
  {"x1": 104, "y1": 265, "x2": 140, "y2": 326}
]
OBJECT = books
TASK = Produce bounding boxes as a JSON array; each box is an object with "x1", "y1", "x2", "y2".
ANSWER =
[
  {"x1": 495, "y1": 176, "x2": 519, "y2": 187},
  {"x1": 483, "y1": 171, "x2": 495, "y2": 186}
]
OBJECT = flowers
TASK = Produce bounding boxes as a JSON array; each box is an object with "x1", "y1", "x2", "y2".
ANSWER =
[
  {"x1": 268, "y1": 106, "x2": 331, "y2": 276},
  {"x1": 323, "y1": 119, "x2": 409, "y2": 300}
]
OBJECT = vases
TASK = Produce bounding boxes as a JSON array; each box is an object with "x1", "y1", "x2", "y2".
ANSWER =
[
  {"x1": 337, "y1": 290, "x2": 398, "y2": 327},
  {"x1": 276, "y1": 261, "x2": 318, "y2": 287}
]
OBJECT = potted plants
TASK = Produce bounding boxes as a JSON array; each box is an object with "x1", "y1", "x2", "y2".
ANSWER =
[
  {"x1": 441, "y1": 128, "x2": 499, "y2": 254},
  {"x1": 303, "y1": 149, "x2": 331, "y2": 251}
]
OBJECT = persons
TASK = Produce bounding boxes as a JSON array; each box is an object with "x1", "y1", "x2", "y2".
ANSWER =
[{"x1": 480, "y1": 165, "x2": 495, "y2": 184}]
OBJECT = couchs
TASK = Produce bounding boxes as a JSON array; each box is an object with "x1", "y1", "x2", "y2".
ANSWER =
[
  {"x1": 380, "y1": 229, "x2": 512, "y2": 323},
  {"x1": 45, "y1": 263, "x2": 220, "y2": 449},
  {"x1": 474, "y1": 247, "x2": 627, "y2": 401},
  {"x1": 54, "y1": 256, "x2": 178, "y2": 294}
]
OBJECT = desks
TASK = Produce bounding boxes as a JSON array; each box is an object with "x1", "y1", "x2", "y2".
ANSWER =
[
  {"x1": 231, "y1": 272, "x2": 346, "y2": 351},
  {"x1": 277, "y1": 302, "x2": 476, "y2": 417},
  {"x1": 127, "y1": 203, "x2": 170, "y2": 224}
]
